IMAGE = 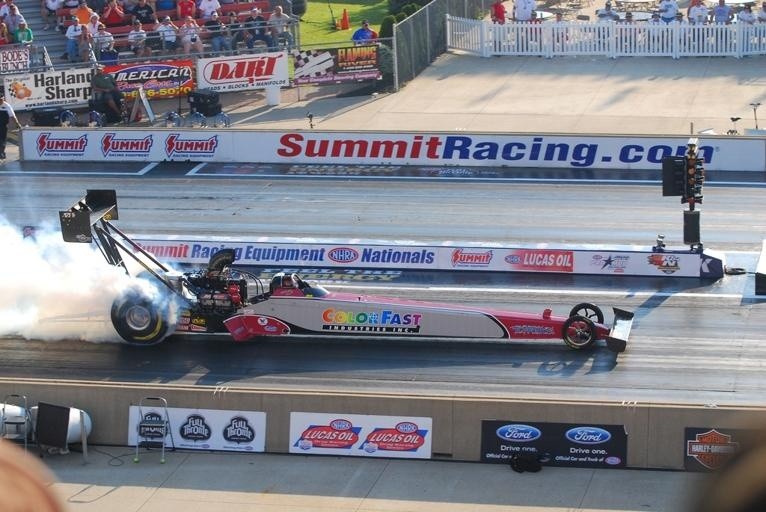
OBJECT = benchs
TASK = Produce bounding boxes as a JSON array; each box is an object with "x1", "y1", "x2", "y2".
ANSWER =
[{"x1": 54, "y1": 2, "x2": 280, "y2": 61}]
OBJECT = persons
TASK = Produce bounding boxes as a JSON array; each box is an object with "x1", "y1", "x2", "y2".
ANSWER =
[{"x1": 491, "y1": 0, "x2": 766, "y2": 57}]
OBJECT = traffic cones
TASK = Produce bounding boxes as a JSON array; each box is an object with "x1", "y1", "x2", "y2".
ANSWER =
[{"x1": 338, "y1": 8, "x2": 352, "y2": 30}]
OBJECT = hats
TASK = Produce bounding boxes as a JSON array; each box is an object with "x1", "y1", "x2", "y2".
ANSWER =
[
  {"x1": 89, "y1": 12, "x2": 100, "y2": 21},
  {"x1": 97, "y1": 63, "x2": 105, "y2": 70},
  {"x1": 362, "y1": 19, "x2": 368, "y2": 26},
  {"x1": 73, "y1": 15, "x2": 79, "y2": 22},
  {"x1": 252, "y1": 7, "x2": 257, "y2": 12},
  {"x1": 212, "y1": 12, "x2": 217, "y2": 17},
  {"x1": 625, "y1": 13, "x2": 634, "y2": 17},
  {"x1": 675, "y1": 12, "x2": 684, "y2": 16}
]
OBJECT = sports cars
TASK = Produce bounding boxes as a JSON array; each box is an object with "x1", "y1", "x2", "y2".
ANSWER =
[{"x1": 54, "y1": 186, "x2": 635, "y2": 354}]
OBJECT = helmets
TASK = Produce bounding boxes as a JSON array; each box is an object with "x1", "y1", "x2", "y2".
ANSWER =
[{"x1": 272, "y1": 273, "x2": 299, "y2": 291}]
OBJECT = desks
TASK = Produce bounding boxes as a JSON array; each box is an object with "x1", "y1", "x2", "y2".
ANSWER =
[{"x1": 505, "y1": 2, "x2": 753, "y2": 42}]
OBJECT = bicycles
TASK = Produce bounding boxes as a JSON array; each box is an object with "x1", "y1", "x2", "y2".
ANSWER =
[{"x1": 727, "y1": 101, "x2": 761, "y2": 136}]
